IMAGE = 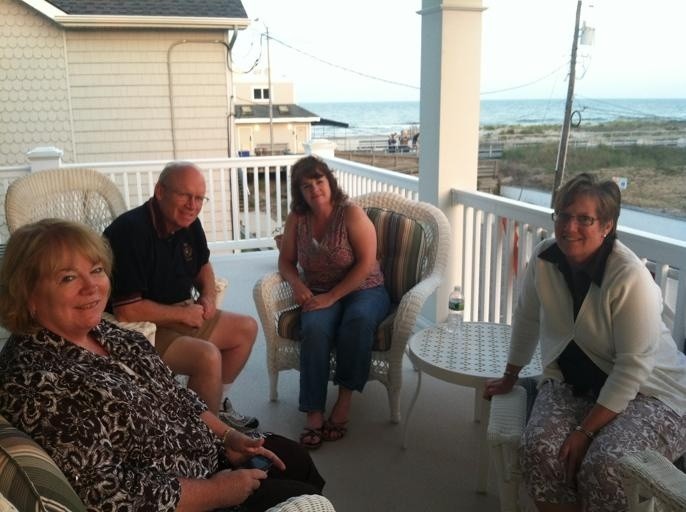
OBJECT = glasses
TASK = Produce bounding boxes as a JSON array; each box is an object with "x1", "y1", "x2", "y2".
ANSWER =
[
  {"x1": 168, "y1": 191, "x2": 209, "y2": 206},
  {"x1": 550, "y1": 212, "x2": 601, "y2": 227}
]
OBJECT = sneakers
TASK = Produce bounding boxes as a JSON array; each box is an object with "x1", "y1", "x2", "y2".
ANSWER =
[{"x1": 219, "y1": 397, "x2": 259, "y2": 431}]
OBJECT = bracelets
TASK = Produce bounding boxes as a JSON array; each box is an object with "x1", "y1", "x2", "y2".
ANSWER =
[
  {"x1": 221, "y1": 428, "x2": 234, "y2": 443},
  {"x1": 575, "y1": 425, "x2": 593, "y2": 441},
  {"x1": 504, "y1": 372, "x2": 518, "y2": 382}
]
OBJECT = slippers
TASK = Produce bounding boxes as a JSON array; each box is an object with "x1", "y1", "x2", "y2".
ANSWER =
[
  {"x1": 299, "y1": 426, "x2": 322, "y2": 449},
  {"x1": 323, "y1": 418, "x2": 350, "y2": 442}
]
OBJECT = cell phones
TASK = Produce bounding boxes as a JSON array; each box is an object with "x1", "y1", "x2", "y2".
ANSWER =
[{"x1": 231, "y1": 453, "x2": 274, "y2": 472}]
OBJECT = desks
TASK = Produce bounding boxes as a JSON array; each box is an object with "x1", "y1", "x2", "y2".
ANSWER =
[{"x1": 398, "y1": 320, "x2": 557, "y2": 497}]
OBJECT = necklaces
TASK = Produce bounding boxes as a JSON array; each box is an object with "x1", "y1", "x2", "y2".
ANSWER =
[{"x1": 485, "y1": 174, "x2": 686, "y2": 511}]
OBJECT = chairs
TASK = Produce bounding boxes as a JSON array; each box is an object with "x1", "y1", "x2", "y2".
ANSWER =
[
  {"x1": 253, "y1": 190, "x2": 453, "y2": 427},
  {"x1": 0, "y1": 411, "x2": 337, "y2": 512},
  {"x1": 2, "y1": 168, "x2": 231, "y2": 362},
  {"x1": 484, "y1": 379, "x2": 659, "y2": 511},
  {"x1": 610, "y1": 448, "x2": 686, "y2": 510}
]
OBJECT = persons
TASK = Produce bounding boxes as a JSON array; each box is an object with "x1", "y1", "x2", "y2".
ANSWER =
[
  {"x1": 277, "y1": 157, "x2": 389, "y2": 450},
  {"x1": 0, "y1": 218, "x2": 323, "y2": 511},
  {"x1": 101, "y1": 163, "x2": 259, "y2": 435}
]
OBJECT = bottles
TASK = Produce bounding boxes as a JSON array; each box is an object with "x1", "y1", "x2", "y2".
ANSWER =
[{"x1": 447, "y1": 285, "x2": 465, "y2": 333}]
